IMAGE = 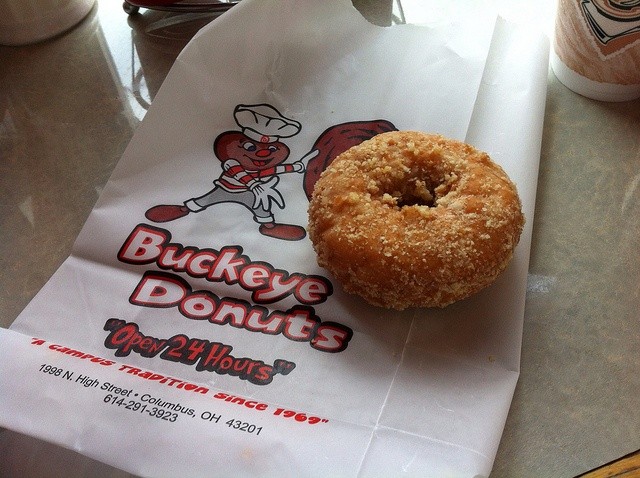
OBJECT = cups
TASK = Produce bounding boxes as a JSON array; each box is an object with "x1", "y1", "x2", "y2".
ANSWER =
[{"x1": 549, "y1": 0, "x2": 640, "y2": 103}]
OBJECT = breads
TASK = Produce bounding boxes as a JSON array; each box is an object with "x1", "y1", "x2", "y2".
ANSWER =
[{"x1": 308, "y1": 131, "x2": 523, "y2": 309}]
